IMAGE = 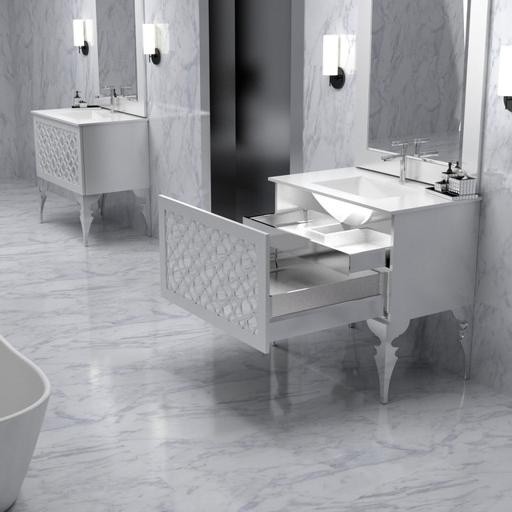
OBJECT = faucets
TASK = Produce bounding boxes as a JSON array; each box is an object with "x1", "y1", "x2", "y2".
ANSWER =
[
  {"x1": 380, "y1": 140, "x2": 408, "y2": 182},
  {"x1": 120, "y1": 86, "x2": 137, "y2": 100},
  {"x1": 412, "y1": 137, "x2": 439, "y2": 158},
  {"x1": 100, "y1": 85, "x2": 116, "y2": 113}
]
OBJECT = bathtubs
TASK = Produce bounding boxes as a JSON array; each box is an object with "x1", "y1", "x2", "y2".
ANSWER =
[{"x1": 0, "y1": 334, "x2": 53, "y2": 512}]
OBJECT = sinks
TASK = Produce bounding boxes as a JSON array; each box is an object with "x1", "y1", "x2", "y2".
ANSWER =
[
  {"x1": 30, "y1": 107, "x2": 146, "y2": 128},
  {"x1": 310, "y1": 176, "x2": 427, "y2": 227}
]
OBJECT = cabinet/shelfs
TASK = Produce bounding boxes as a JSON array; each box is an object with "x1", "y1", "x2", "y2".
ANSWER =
[
  {"x1": 31, "y1": 107, "x2": 150, "y2": 247},
  {"x1": 157, "y1": 168, "x2": 482, "y2": 405}
]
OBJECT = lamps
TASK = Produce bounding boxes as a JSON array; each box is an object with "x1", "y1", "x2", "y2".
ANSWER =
[
  {"x1": 73, "y1": 18, "x2": 88, "y2": 55},
  {"x1": 322, "y1": 34, "x2": 345, "y2": 88},
  {"x1": 497, "y1": 46, "x2": 512, "y2": 113},
  {"x1": 142, "y1": 24, "x2": 160, "y2": 64}
]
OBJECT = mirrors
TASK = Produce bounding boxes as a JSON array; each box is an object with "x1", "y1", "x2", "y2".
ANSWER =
[
  {"x1": 88, "y1": 0, "x2": 147, "y2": 117},
  {"x1": 353, "y1": 0, "x2": 492, "y2": 192}
]
